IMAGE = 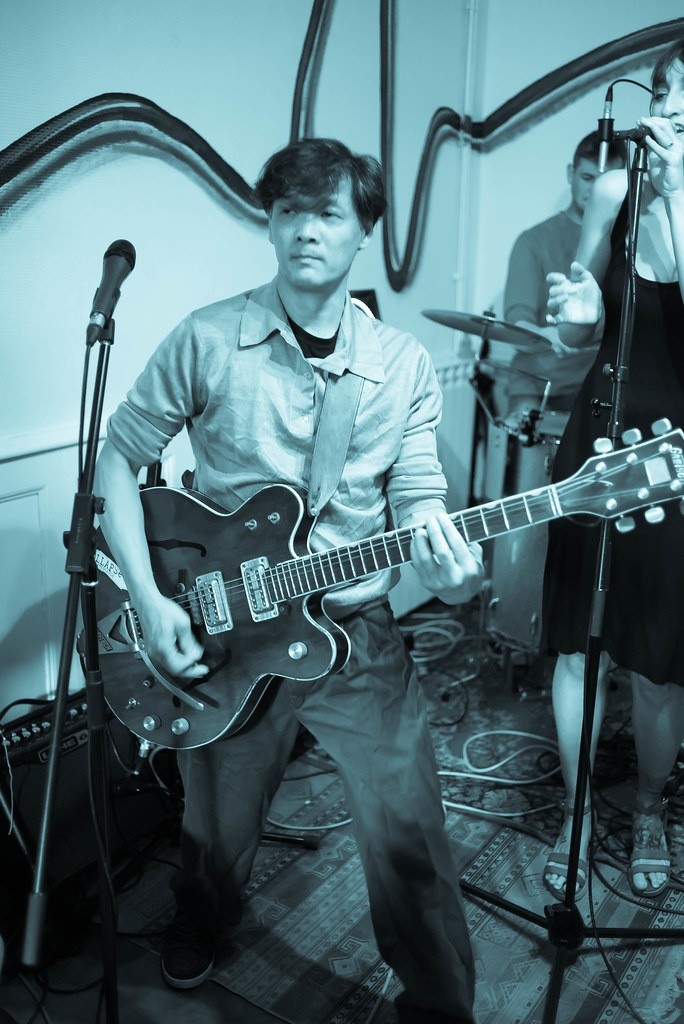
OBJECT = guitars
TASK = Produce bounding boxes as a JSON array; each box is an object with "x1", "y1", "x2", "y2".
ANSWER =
[{"x1": 79, "y1": 416, "x2": 683, "y2": 752}]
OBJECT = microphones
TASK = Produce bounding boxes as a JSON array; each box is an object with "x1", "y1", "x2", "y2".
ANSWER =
[
  {"x1": 598, "y1": 86, "x2": 614, "y2": 172},
  {"x1": 612, "y1": 127, "x2": 655, "y2": 141},
  {"x1": 84, "y1": 239, "x2": 136, "y2": 347}
]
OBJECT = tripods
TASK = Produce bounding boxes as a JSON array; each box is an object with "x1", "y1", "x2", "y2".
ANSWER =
[{"x1": 457, "y1": 139, "x2": 684, "y2": 1024}]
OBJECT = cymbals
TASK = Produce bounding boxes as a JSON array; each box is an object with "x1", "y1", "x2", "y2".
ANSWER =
[{"x1": 422, "y1": 307, "x2": 552, "y2": 351}]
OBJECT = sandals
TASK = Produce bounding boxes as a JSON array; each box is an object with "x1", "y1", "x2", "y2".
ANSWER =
[
  {"x1": 543, "y1": 798, "x2": 597, "y2": 903},
  {"x1": 629, "y1": 790, "x2": 671, "y2": 897}
]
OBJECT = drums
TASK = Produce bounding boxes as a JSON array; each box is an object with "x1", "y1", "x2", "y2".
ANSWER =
[{"x1": 485, "y1": 407, "x2": 577, "y2": 508}]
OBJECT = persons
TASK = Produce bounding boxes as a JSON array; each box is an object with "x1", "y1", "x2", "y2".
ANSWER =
[
  {"x1": 503, "y1": 40, "x2": 684, "y2": 905},
  {"x1": 91, "y1": 139, "x2": 486, "y2": 1024}
]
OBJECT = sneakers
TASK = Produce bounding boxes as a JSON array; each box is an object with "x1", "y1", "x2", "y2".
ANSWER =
[{"x1": 160, "y1": 912, "x2": 219, "y2": 990}]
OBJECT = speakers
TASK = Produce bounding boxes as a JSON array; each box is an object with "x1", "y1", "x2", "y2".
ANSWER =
[{"x1": 1, "y1": 688, "x2": 185, "y2": 970}]
